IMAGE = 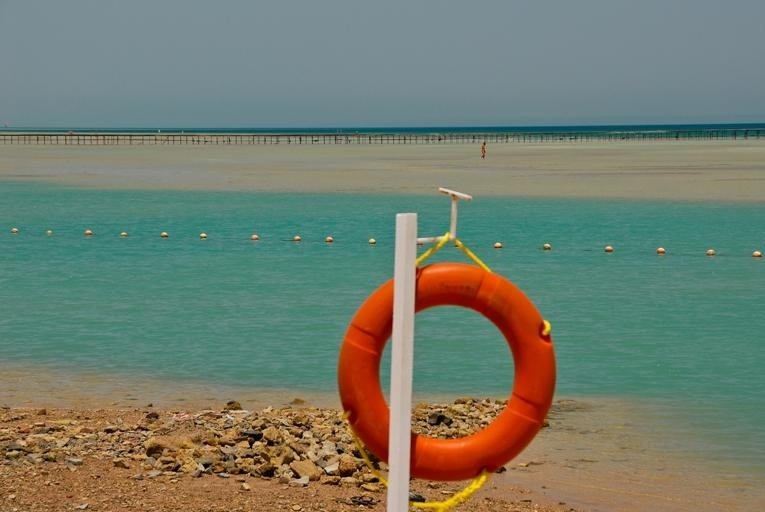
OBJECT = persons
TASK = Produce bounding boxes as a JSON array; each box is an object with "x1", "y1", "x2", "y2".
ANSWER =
[{"x1": 480, "y1": 142, "x2": 487, "y2": 160}]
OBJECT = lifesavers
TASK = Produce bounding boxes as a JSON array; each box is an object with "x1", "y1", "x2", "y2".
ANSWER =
[{"x1": 339, "y1": 262, "x2": 555, "y2": 483}]
